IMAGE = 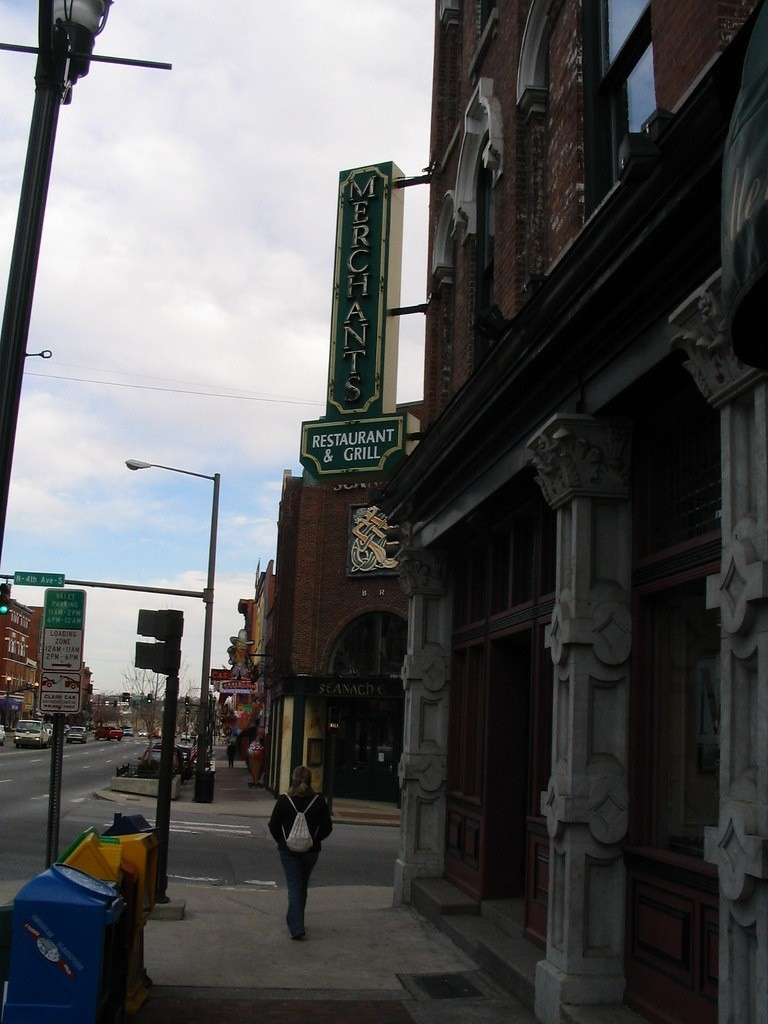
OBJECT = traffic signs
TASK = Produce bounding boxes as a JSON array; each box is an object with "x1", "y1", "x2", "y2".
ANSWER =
[{"x1": 42, "y1": 628, "x2": 83, "y2": 670}]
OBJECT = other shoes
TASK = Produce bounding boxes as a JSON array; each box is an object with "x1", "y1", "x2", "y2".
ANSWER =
[{"x1": 289, "y1": 931, "x2": 305, "y2": 938}]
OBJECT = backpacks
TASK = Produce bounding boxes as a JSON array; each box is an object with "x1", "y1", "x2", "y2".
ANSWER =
[{"x1": 279, "y1": 792, "x2": 319, "y2": 852}]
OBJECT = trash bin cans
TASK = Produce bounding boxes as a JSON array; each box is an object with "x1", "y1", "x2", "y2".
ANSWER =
[
  {"x1": 0, "y1": 810, "x2": 162, "y2": 1024},
  {"x1": 194, "y1": 770, "x2": 217, "y2": 803}
]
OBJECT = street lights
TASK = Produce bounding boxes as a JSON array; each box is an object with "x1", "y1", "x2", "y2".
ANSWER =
[
  {"x1": 1, "y1": 0, "x2": 172, "y2": 546},
  {"x1": 124, "y1": 458, "x2": 222, "y2": 803}
]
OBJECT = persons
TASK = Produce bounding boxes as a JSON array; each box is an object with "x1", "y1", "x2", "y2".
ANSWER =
[
  {"x1": 267, "y1": 764, "x2": 333, "y2": 940},
  {"x1": 226, "y1": 741, "x2": 236, "y2": 767}
]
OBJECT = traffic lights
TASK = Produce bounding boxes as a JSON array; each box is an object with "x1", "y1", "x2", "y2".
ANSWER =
[
  {"x1": 0, "y1": 583, "x2": 11, "y2": 615},
  {"x1": 184, "y1": 697, "x2": 190, "y2": 706},
  {"x1": 147, "y1": 694, "x2": 152, "y2": 703},
  {"x1": 123, "y1": 693, "x2": 130, "y2": 702},
  {"x1": 185, "y1": 709, "x2": 190, "y2": 714}
]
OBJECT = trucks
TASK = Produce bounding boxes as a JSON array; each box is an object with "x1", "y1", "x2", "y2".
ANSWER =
[{"x1": 14, "y1": 718, "x2": 53, "y2": 748}]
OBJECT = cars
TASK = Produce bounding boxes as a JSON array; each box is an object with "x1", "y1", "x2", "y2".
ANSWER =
[
  {"x1": 63, "y1": 724, "x2": 87, "y2": 744},
  {"x1": 122, "y1": 726, "x2": 134, "y2": 737},
  {"x1": 95, "y1": 726, "x2": 123, "y2": 741},
  {"x1": 139, "y1": 728, "x2": 160, "y2": 738},
  {"x1": 181, "y1": 731, "x2": 192, "y2": 742},
  {"x1": 138, "y1": 742, "x2": 192, "y2": 776},
  {"x1": 0, "y1": 724, "x2": 6, "y2": 746}
]
